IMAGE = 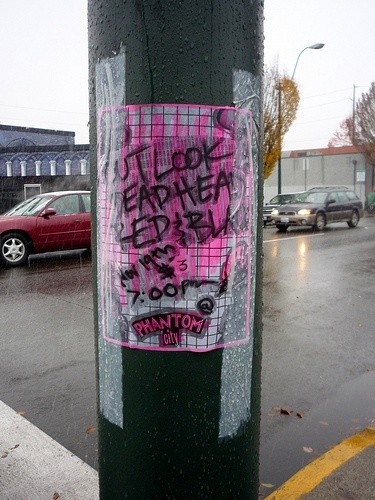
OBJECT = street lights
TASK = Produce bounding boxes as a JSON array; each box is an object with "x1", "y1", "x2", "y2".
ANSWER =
[{"x1": 278, "y1": 42, "x2": 324, "y2": 194}]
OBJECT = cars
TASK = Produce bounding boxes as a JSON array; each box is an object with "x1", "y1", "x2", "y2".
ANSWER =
[
  {"x1": 271, "y1": 185, "x2": 365, "y2": 232},
  {"x1": 0, "y1": 191, "x2": 94, "y2": 266},
  {"x1": 261, "y1": 191, "x2": 305, "y2": 226}
]
